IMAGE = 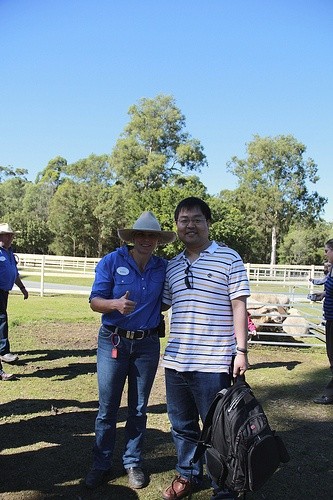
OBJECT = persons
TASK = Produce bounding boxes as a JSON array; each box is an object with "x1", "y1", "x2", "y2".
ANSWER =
[
  {"x1": 305, "y1": 239, "x2": 333, "y2": 404},
  {"x1": 84, "y1": 211, "x2": 228, "y2": 490},
  {"x1": 234, "y1": 315, "x2": 256, "y2": 342},
  {"x1": 161, "y1": 197, "x2": 249, "y2": 500},
  {"x1": 0, "y1": 222, "x2": 28, "y2": 362},
  {"x1": 0, "y1": 364, "x2": 15, "y2": 380}
]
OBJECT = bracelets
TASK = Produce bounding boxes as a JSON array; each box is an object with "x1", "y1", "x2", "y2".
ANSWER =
[{"x1": 237, "y1": 347, "x2": 248, "y2": 354}]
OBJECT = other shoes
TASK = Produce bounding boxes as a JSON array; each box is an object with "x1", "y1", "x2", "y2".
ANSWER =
[
  {"x1": 0, "y1": 372, "x2": 14, "y2": 381},
  {"x1": 313, "y1": 394, "x2": 333, "y2": 404},
  {"x1": 86, "y1": 468, "x2": 108, "y2": 490},
  {"x1": 0, "y1": 353, "x2": 19, "y2": 363},
  {"x1": 127, "y1": 467, "x2": 146, "y2": 488},
  {"x1": 326, "y1": 378, "x2": 333, "y2": 388}
]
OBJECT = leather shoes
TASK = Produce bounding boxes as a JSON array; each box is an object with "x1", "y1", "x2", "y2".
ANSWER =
[{"x1": 163, "y1": 476, "x2": 196, "y2": 500}]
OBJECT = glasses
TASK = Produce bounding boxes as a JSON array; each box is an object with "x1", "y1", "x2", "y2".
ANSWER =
[{"x1": 185, "y1": 264, "x2": 193, "y2": 289}]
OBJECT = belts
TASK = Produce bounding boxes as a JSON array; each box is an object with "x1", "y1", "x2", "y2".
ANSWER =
[{"x1": 104, "y1": 325, "x2": 158, "y2": 340}]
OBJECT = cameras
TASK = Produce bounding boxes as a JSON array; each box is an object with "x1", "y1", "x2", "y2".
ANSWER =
[{"x1": 307, "y1": 294, "x2": 317, "y2": 301}]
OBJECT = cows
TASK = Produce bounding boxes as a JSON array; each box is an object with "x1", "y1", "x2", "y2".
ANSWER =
[{"x1": 247, "y1": 296, "x2": 308, "y2": 339}]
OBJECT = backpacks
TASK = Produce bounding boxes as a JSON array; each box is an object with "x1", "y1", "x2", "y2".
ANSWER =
[{"x1": 189, "y1": 375, "x2": 290, "y2": 500}]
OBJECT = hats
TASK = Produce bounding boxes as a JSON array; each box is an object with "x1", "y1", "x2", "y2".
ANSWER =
[
  {"x1": 118, "y1": 211, "x2": 176, "y2": 244},
  {"x1": 0, "y1": 223, "x2": 21, "y2": 235}
]
